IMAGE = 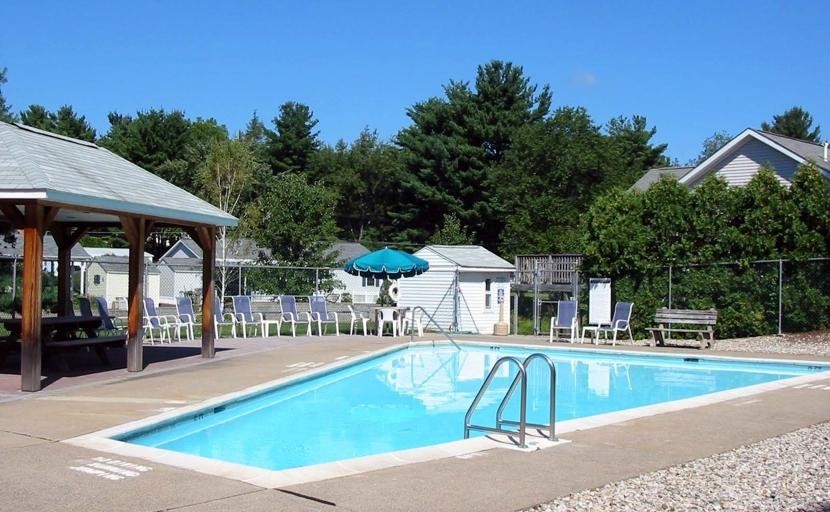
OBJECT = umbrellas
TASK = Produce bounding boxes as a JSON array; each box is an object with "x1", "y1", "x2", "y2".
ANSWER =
[{"x1": 344, "y1": 246, "x2": 429, "y2": 333}]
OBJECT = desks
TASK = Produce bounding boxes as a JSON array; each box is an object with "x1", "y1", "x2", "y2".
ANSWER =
[{"x1": 2, "y1": 316, "x2": 102, "y2": 375}]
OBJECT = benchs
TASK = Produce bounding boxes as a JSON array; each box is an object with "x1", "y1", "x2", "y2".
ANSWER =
[
  {"x1": 43, "y1": 335, "x2": 126, "y2": 372},
  {"x1": 643, "y1": 306, "x2": 718, "y2": 350},
  {"x1": 1, "y1": 335, "x2": 21, "y2": 367}
]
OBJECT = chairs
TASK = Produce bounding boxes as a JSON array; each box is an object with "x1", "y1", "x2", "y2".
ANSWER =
[
  {"x1": 142, "y1": 295, "x2": 202, "y2": 346},
  {"x1": 214, "y1": 295, "x2": 340, "y2": 338},
  {"x1": 347, "y1": 305, "x2": 424, "y2": 337},
  {"x1": 77, "y1": 296, "x2": 128, "y2": 344},
  {"x1": 549, "y1": 299, "x2": 635, "y2": 346}
]
